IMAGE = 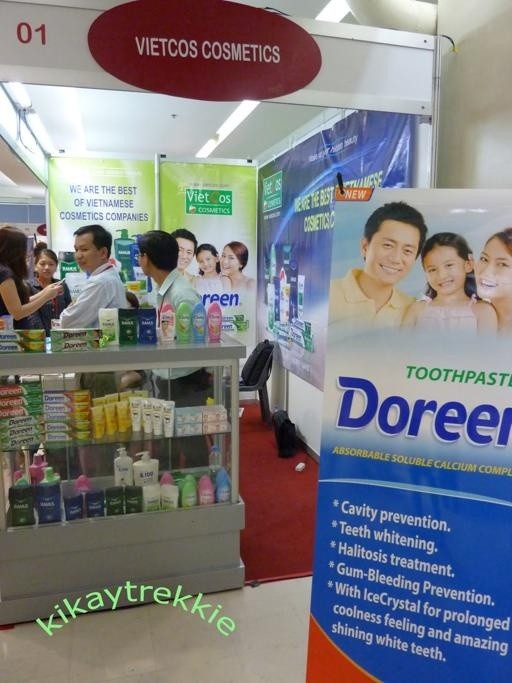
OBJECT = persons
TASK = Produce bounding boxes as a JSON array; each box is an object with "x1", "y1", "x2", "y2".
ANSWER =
[
  {"x1": 171, "y1": 229, "x2": 198, "y2": 289},
  {"x1": 401, "y1": 232, "x2": 498, "y2": 339},
  {"x1": 28, "y1": 242, "x2": 48, "y2": 278},
  {"x1": 196, "y1": 243, "x2": 231, "y2": 292},
  {"x1": 27, "y1": 248, "x2": 73, "y2": 336},
  {"x1": 477, "y1": 228, "x2": 512, "y2": 338},
  {"x1": 134, "y1": 229, "x2": 203, "y2": 317},
  {"x1": 220, "y1": 241, "x2": 256, "y2": 295},
  {"x1": 329, "y1": 202, "x2": 427, "y2": 340},
  {"x1": 60, "y1": 225, "x2": 130, "y2": 328},
  {"x1": 0, "y1": 226, "x2": 65, "y2": 330}
]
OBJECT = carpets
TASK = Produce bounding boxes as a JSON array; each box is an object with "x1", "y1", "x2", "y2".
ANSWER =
[{"x1": 240, "y1": 401, "x2": 318, "y2": 584}]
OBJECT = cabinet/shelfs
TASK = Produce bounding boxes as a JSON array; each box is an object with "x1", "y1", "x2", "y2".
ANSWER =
[{"x1": 0, "y1": 337, "x2": 244, "y2": 623}]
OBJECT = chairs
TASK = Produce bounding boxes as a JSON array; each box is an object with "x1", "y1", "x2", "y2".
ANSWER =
[{"x1": 238, "y1": 351, "x2": 273, "y2": 421}]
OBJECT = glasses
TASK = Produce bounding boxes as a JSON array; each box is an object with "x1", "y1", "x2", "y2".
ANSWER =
[{"x1": 134, "y1": 253, "x2": 145, "y2": 258}]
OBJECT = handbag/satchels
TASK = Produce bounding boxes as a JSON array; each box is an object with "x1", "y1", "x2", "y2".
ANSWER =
[{"x1": 267, "y1": 410, "x2": 297, "y2": 458}]
